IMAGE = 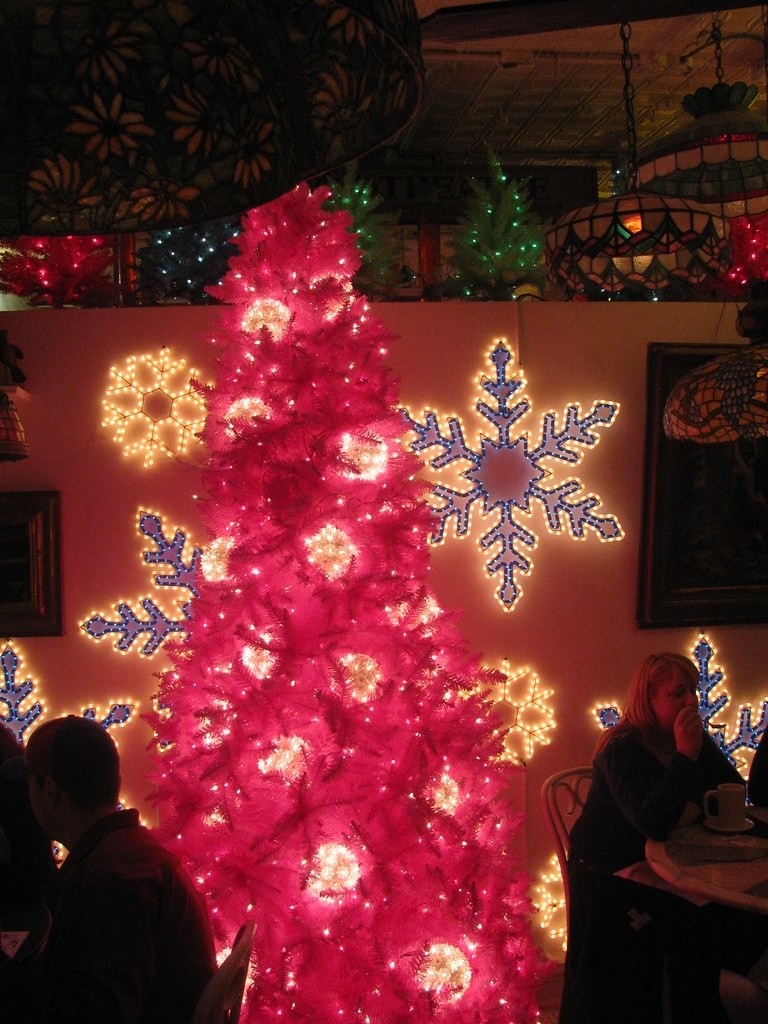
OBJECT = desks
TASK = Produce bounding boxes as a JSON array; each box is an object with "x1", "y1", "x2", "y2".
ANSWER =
[{"x1": 642, "y1": 806, "x2": 768, "y2": 911}]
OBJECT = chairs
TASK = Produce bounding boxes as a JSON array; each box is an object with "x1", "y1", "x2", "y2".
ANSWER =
[
  {"x1": 195, "y1": 921, "x2": 258, "y2": 1024},
  {"x1": 539, "y1": 764, "x2": 720, "y2": 1024}
]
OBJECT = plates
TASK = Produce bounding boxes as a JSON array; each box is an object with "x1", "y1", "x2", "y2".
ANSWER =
[{"x1": 704, "y1": 814, "x2": 754, "y2": 832}]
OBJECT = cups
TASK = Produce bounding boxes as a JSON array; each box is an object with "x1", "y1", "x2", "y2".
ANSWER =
[{"x1": 703, "y1": 784, "x2": 745, "y2": 829}]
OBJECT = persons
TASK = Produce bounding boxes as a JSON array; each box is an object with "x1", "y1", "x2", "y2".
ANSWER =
[
  {"x1": 0, "y1": 715, "x2": 219, "y2": 1024},
  {"x1": 557, "y1": 651, "x2": 768, "y2": 1024}
]
